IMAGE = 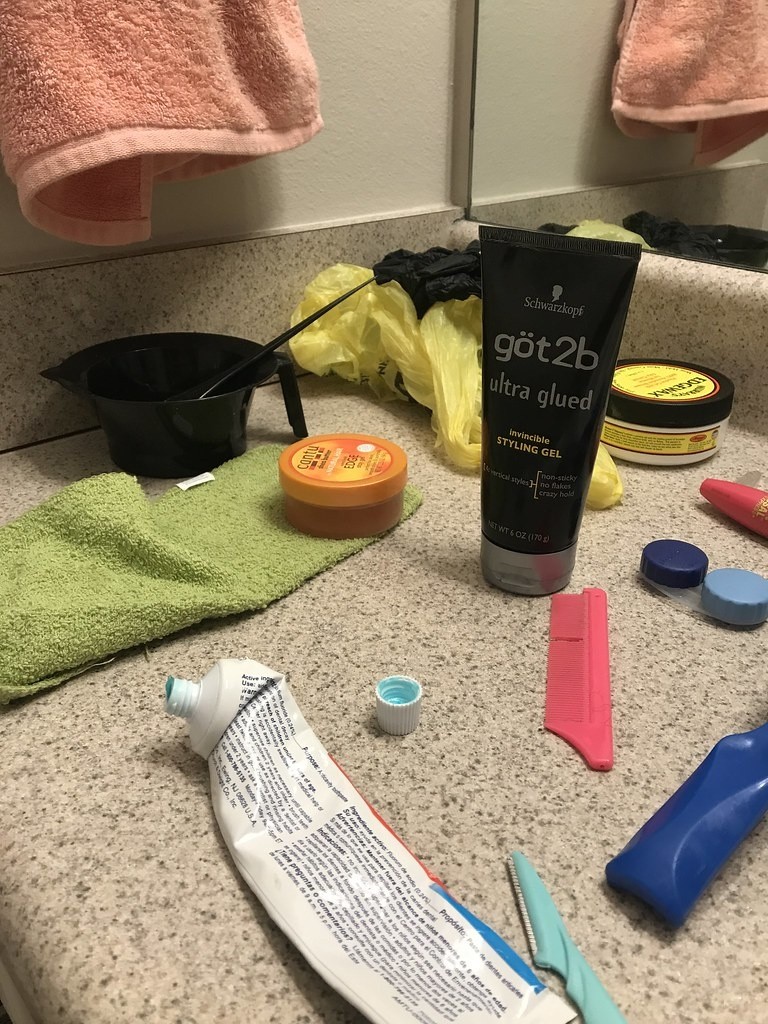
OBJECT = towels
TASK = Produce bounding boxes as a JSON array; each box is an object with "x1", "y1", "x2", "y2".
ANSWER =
[
  {"x1": 0, "y1": 0, "x2": 327, "y2": 248},
  {"x1": 0, "y1": 441, "x2": 424, "y2": 707},
  {"x1": 609, "y1": 0, "x2": 768, "y2": 170}
]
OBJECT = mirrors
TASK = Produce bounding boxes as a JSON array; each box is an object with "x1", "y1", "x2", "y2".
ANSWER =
[{"x1": 464, "y1": 1, "x2": 768, "y2": 274}]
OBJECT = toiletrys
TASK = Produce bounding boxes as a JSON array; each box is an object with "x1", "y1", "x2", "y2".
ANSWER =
[{"x1": 164, "y1": 656, "x2": 580, "y2": 1024}]
